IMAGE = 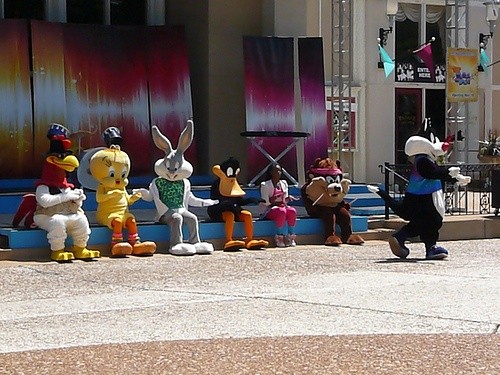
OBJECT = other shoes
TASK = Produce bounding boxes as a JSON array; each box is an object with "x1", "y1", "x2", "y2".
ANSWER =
[
  {"x1": 274, "y1": 234, "x2": 285, "y2": 247},
  {"x1": 287, "y1": 233, "x2": 296, "y2": 247}
]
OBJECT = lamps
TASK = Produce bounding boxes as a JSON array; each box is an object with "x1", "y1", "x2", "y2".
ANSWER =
[
  {"x1": 478, "y1": 0, "x2": 500, "y2": 72},
  {"x1": 377, "y1": 0, "x2": 399, "y2": 69}
]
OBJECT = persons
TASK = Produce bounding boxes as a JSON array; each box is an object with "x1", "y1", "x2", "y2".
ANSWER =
[{"x1": 260, "y1": 164, "x2": 300, "y2": 247}]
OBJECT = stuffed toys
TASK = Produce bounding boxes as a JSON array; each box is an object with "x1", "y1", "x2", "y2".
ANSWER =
[
  {"x1": 89, "y1": 144, "x2": 156, "y2": 256},
  {"x1": 368, "y1": 117, "x2": 471, "y2": 260},
  {"x1": 208, "y1": 159, "x2": 269, "y2": 251},
  {"x1": 133, "y1": 120, "x2": 219, "y2": 255},
  {"x1": 301, "y1": 158, "x2": 365, "y2": 246},
  {"x1": 13, "y1": 123, "x2": 101, "y2": 261}
]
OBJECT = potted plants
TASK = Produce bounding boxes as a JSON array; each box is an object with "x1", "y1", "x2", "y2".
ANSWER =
[{"x1": 475, "y1": 128, "x2": 500, "y2": 163}]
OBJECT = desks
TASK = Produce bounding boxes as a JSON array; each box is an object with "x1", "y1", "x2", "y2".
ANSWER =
[{"x1": 240, "y1": 131, "x2": 311, "y2": 187}]
